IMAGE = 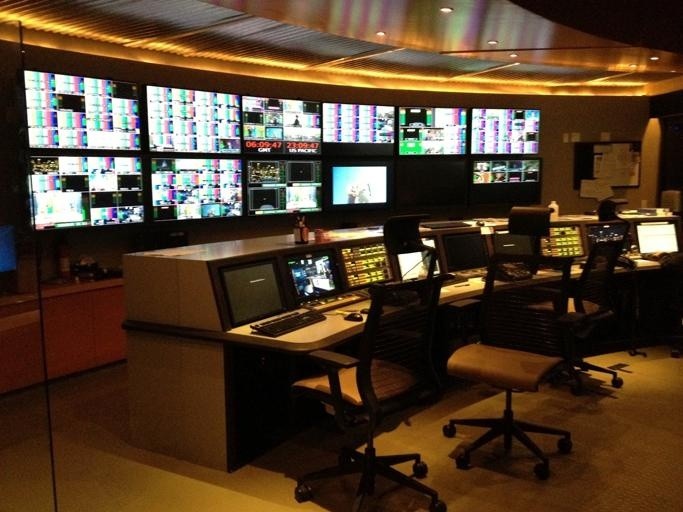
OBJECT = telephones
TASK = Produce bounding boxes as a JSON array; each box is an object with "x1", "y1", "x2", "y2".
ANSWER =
[{"x1": 497, "y1": 259, "x2": 538, "y2": 281}]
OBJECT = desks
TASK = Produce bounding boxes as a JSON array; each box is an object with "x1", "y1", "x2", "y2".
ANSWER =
[{"x1": 121, "y1": 210, "x2": 683, "y2": 474}]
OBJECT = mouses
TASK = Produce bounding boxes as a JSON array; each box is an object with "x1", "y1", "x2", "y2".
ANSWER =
[
  {"x1": 360, "y1": 308, "x2": 369, "y2": 313},
  {"x1": 344, "y1": 313, "x2": 363, "y2": 322}
]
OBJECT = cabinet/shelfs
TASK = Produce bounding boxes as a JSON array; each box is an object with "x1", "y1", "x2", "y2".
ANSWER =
[{"x1": 1, "y1": 280, "x2": 129, "y2": 398}]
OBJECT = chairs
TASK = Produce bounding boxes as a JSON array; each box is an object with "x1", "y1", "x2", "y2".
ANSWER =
[
  {"x1": 519, "y1": 240, "x2": 625, "y2": 401},
  {"x1": 289, "y1": 273, "x2": 458, "y2": 512},
  {"x1": 443, "y1": 254, "x2": 574, "y2": 482}
]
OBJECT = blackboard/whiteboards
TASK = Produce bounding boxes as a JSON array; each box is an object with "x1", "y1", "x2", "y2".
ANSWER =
[{"x1": 571, "y1": 139, "x2": 642, "y2": 191}]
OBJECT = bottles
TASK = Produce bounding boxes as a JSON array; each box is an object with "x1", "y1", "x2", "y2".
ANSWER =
[
  {"x1": 294, "y1": 214, "x2": 308, "y2": 244},
  {"x1": 549, "y1": 200, "x2": 559, "y2": 221}
]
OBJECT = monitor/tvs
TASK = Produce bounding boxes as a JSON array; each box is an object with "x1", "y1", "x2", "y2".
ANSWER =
[
  {"x1": 396, "y1": 158, "x2": 466, "y2": 209},
  {"x1": 585, "y1": 220, "x2": 632, "y2": 260},
  {"x1": 143, "y1": 82, "x2": 242, "y2": 157},
  {"x1": 633, "y1": 220, "x2": 680, "y2": 257},
  {"x1": 440, "y1": 231, "x2": 492, "y2": 273},
  {"x1": 468, "y1": 157, "x2": 541, "y2": 186},
  {"x1": 330, "y1": 163, "x2": 391, "y2": 210},
  {"x1": 218, "y1": 256, "x2": 288, "y2": 328},
  {"x1": 21, "y1": 67, "x2": 148, "y2": 156},
  {"x1": 470, "y1": 107, "x2": 541, "y2": 157},
  {"x1": 244, "y1": 159, "x2": 326, "y2": 215},
  {"x1": 397, "y1": 106, "x2": 469, "y2": 156},
  {"x1": 242, "y1": 97, "x2": 321, "y2": 158},
  {"x1": 491, "y1": 229, "x2": 536, "y2": 265},
  {"x1": 23, "y1": 155, "x2": 148, "y2": 233},
  {"x1": 394, "y1": 238, "x2": 441, "y2": 283},
  {"x1": 539, "y1": 224, "x2": 585, "y2": 260},
  {"x1": 322, "y1": 102, "x2": 396, "y2": 154},
  {"x1": 340, "y1": 241, "x2": 394, "y2": 289},
  {"x1": 283, "y1": 247, "x2": 344, "y2": 299},
  {"x1": 146, "y1": 156, "x2": 245, "y2": 223}
]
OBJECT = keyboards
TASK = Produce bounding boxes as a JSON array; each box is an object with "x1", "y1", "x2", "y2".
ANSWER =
[
  {"x1": 250, "y1": 307, "x2": 327, "y2": 338},
  {"x1": 304, "y1": 292, "x2": 371, "y2": 312},
  {"x1": 427, "y1": 273, "x2": 469, "y2": 287},
  {"x1": 352, "y1": 288, "x2": 371, "y2": 299}
]
